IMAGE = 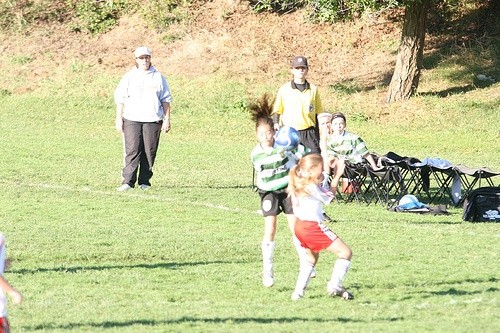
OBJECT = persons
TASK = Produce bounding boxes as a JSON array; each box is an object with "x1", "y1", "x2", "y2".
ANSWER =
[
  {"x1": 248, "y1": 92, "x2": 315, "y2": 288},
  {"x1": 113, "y1": 46, "x2": 172, "y2": 192},
  {"x1": 315, "y1": 112, "x2": 384, "y2": 192},
  {"x1": 0, "y1": 231, "x2": 23, "y2": 333},
  {"x1": 284, "y1": 152, "x2": 354, "y2": 300},
  {"x1": 270, "y1": 56, "x2": 333, "y2": 222}
]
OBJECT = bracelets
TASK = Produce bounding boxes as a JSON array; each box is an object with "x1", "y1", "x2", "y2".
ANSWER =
[{"x1": 164, "y1": 119, "x2": 170, "y2": 120}]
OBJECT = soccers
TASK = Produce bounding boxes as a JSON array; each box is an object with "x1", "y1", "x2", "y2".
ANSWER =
[
  {"x1": 274, "y1": 125, "x2": 302, "y2": 151},
  {"x1": 399, "y1": 195, "x2": 419, "y2": 205}
]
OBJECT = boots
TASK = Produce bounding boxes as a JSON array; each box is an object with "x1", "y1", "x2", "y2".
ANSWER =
[
  {"x1": 290, "y1": 262, "x2": 315, "y2": 300},
  {"x1": 292, "y1": 236, "x2": 316, "y2": 278},
  {"x1": 261, "y1": 240, "x2": 276, "y2": 286},
  {"x1": 327, "y1": 258, "x2": 354, "y2": 299}
]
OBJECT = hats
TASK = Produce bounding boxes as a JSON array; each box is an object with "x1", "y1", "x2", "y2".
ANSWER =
[
  {"x1": 135, "y1": 45, "x2": 151, "y2": 58},
  {"x1": 291, "y1": 56, "x2": 308, "y2": 68},
  {"x1": 331, "y1": 111, "x2": 346, "y2": 122}
]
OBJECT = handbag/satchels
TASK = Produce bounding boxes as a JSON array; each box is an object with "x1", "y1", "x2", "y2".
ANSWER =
[{"x1": 461, "y1": 186, "x2": 500, "y2": 222}]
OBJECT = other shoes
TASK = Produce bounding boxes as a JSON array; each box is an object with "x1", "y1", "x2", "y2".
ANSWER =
[
  {"x1": 116, "y1": 183, "x2": 132, "y2": 191},
  {"x1": 139, "y1": 183, "x2": 151, "y2": 190}
]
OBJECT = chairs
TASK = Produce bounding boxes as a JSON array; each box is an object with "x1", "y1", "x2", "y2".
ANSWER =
[{"x1": 332, "y1": 151, "x2": 500, "y2": 211}]
